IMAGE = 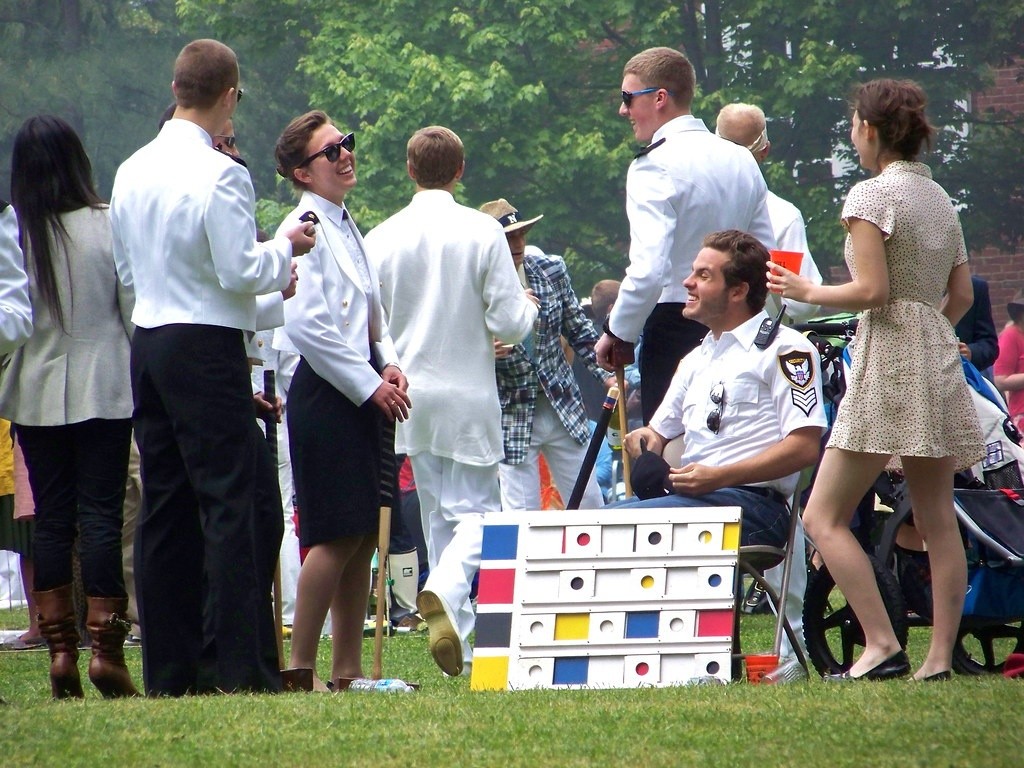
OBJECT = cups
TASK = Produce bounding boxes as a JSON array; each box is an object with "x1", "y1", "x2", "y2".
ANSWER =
[
  {"x1": 767, "y1": 248, "x2": 804, "y2": 292},
  {"x1": 745, "y1": 653, "x2": 778, "y2": 685}
]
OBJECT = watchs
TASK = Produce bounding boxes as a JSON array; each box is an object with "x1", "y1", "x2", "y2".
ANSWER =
[{"x1": 601, "y1": 315, "x2": 617, "y2": 339}]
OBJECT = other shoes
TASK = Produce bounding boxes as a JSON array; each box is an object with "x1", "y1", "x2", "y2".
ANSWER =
[
  {"x1": 912, "y1": 649, "x2": 951, "y2": 683},
  {"x1": 827, "y1": 650, "x2": 912, "y2": 680},
  {"x1": 8, "y1": 634, "x2": 44, "y2": 648},
  {"x1": 417, "y1": 589, "x2": 464, "y2": 678},
  {"x1": 122, "y1": 632, "x2": 142, "y2": 647}
]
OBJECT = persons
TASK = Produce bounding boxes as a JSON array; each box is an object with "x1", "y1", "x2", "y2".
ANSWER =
[
  {"x1": 108, "y1": 39, "x2": 316, "y2": 695},
  {"x1": 0, "y1": 101, "x2": 1024, "y2": 700},
  {"x1": 595, "y1": 46, "x2": 774, "y2": 426},
  {"x1": 765, "y1": 78, "x2": 981, "y2": 681}
]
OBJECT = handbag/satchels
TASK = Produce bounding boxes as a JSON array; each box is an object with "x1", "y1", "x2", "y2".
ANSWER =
[{"x1": 742, "y1": 576, "x2": 776, "y2": 614}]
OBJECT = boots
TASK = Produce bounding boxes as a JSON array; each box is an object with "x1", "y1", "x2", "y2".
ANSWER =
[{"x1": 32, "y1": 584, "x2": 144, "y2": 699}]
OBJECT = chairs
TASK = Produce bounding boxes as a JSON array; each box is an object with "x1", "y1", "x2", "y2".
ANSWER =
[{"x1": 663, "y1": 433, "x2": 810, "y2": 684}]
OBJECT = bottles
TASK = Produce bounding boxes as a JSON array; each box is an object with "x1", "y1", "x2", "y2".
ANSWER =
[{"x1": 607, "y1": 382, "x2": 628, "y2": 452}]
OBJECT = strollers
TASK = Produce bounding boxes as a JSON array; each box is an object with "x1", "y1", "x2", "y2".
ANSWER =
[{"x1": 798, "y1": 321, "x2": 1024, "y2": 679}]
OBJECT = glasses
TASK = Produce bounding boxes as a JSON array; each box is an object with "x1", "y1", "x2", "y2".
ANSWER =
[
  {"x1": 237, "y1": 90, "x2": 243, "y2": 102},
  {"x1": 218, "y1": 135, "x2": 235, "y2": 147},
  {"x1": 621, "y1": 87, "x2": 674, "y2": 107},
  {"x1": 296, "y1": 131, "x2": 355, "y2": 168}
]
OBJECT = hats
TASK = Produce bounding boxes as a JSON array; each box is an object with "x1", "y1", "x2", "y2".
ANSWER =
[
  {"x1": 478, "y1": 198, "x2": 544, "y2": 235},
  {"x1": 1007, "y1": 287, "x2": 1024, "y2": 323}
]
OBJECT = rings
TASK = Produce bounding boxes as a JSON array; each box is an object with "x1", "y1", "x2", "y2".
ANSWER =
[{"x1": 391, "y1": 400, "x2": 396, "y2": 406}]
